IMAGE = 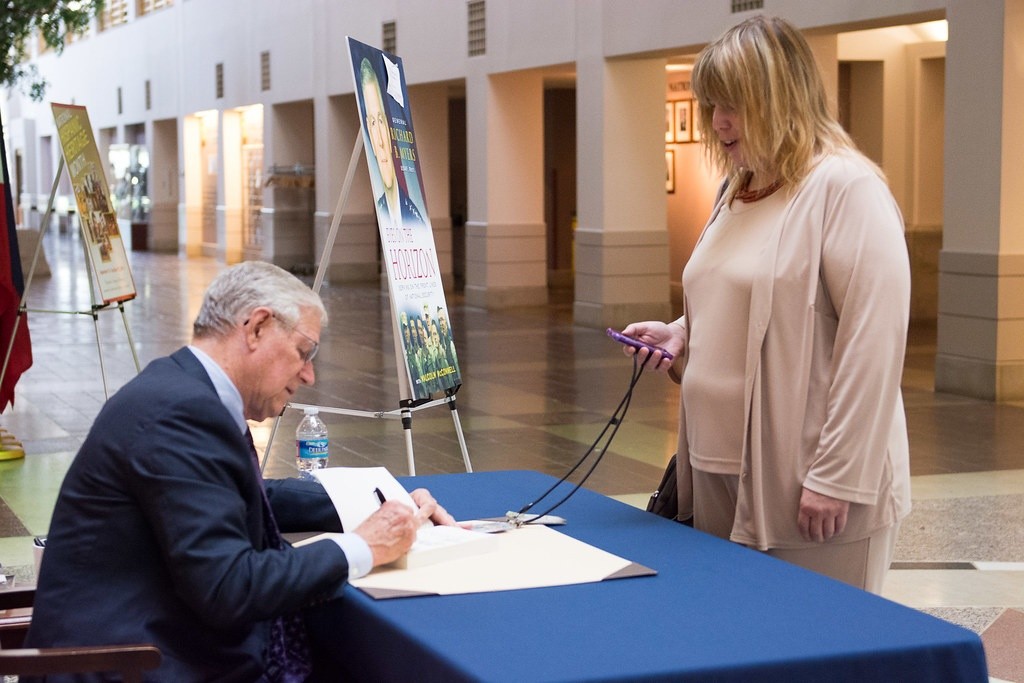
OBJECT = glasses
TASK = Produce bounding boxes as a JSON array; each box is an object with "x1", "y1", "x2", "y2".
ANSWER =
[{"x1": 244, "y1": 315, "x2": 320, "y2": 363}]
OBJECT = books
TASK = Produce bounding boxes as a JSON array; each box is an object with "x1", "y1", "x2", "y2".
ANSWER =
[{"x1": 311, "y1": 465, "x2": 488, "y2": 594}]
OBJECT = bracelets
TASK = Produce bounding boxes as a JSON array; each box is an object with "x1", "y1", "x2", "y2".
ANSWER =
[{"x1": 672, "y1": 320, "x2": 685, "y2": 330}]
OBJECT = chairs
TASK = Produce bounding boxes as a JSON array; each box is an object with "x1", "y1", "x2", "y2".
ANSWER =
[{"x1": 0, "y1": 589, "x2": 164, "y2": 683}]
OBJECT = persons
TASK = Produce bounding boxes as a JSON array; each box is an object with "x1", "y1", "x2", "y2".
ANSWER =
[
  {"x1": 18, "y1": 260, "x2": 456, "y2": 683},
  {"x1": 620, "y1": 14, "x2": 913, "y2": 596},
  {"x1": 401, "y1": 306, "x2": 459, "y2": 399},
  {"x1": 360, "y1": 58, "x2": 424, "y2": 225}
]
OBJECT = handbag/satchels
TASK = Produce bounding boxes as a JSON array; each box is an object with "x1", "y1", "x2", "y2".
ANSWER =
[{"x1": 646, "y1": 455, "x2": 678, "y2": 521}]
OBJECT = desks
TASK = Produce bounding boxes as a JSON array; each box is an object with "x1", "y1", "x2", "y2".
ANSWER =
[{"x1": 282, "y1": 469, "x2": 987, "y2": 683}]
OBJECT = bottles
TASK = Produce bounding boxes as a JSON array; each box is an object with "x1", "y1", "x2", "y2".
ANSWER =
[{"x1": 294, "y1": 406, "x2": 328, "y2": 479}]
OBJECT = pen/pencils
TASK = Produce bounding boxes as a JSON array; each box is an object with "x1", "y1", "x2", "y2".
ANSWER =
[{"x1": 373, "y1": 487, "x2": 386, "y2": 504}]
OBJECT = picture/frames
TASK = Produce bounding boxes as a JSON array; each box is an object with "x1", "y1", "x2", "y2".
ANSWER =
[
  {"x1": 665, "y1": 149, "x2": 675, "y2": 194},
  {"x1": 664, "y1": 99, "x2": 703, "y2": 144}
]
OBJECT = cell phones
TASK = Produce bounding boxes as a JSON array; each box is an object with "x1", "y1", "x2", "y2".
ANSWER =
[{"x1": 606, "y1": 328, "x2": 673, "y2": 360}]
